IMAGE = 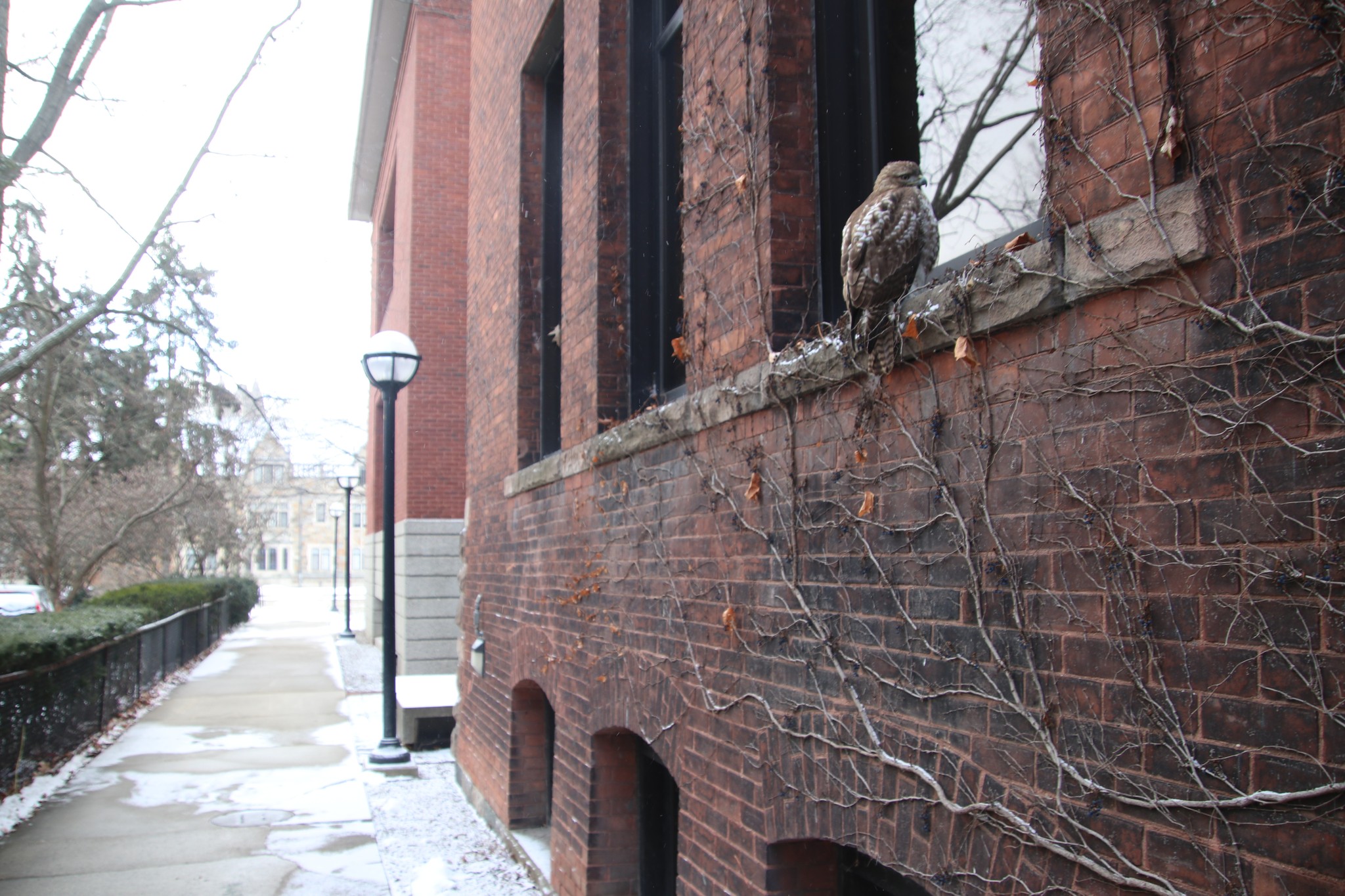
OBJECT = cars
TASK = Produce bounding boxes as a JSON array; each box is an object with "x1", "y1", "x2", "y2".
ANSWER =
[{"x1": 0, "y1": 583, "x2": 65, "y2": 617}]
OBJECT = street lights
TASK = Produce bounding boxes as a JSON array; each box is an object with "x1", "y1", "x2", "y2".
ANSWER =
[
  {"x1": 359, "y1": 330, "x2": 411, "y2": 764},
  {"x1": 336, "y1": 465, "x2": 362, "y2": 639},
  {"x1": 328, "y1": 501, "x2": 343, "y2": 612}
]
